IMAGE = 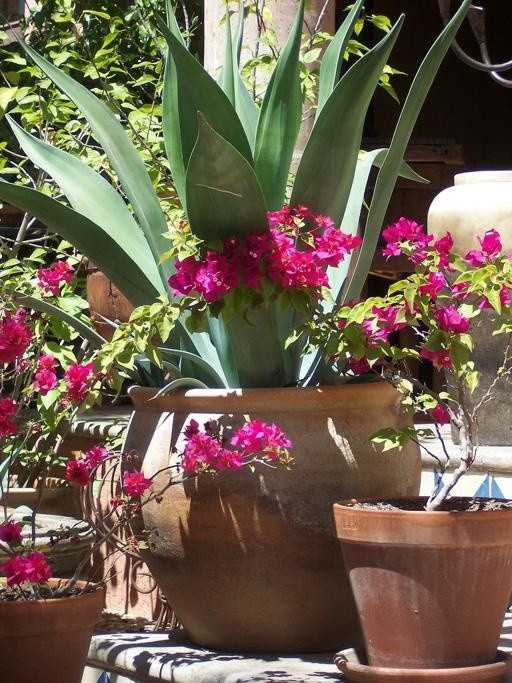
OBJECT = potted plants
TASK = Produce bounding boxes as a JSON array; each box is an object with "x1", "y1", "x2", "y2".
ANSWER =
[{"x1": 0, "y1": 0, "x2": 485, "y2": 657}]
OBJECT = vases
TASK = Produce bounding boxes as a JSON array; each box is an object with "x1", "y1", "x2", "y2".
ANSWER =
[
  {"x1": 1, "y1": 576, "x2": 104, "y2": 682},
  {"x1": 330, "y1": 493, "x2": 511, "y2": 682}
]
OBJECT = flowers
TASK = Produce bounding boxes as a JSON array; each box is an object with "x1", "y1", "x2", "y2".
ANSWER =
[{"x1": 1, "y1": 201, "x2": 509, "y2": 598}]
